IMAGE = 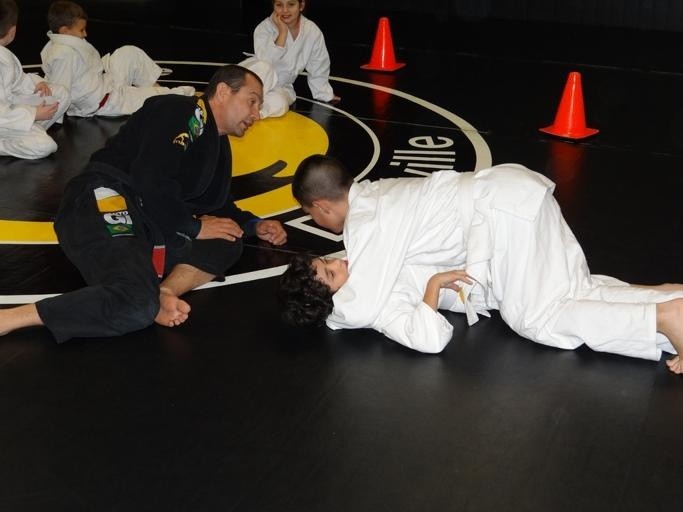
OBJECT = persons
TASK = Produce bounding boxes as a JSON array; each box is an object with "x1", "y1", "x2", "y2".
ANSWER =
[
  {"x1": 292, "y1": 155, "x2": 680, "y2": 373},
  {"x1": 234, "y1": 0, "x2": 338, "y2": 119},
  {"x1": 40, "y1": 2, "x2": 207, "y2": 117},
  {"x1": 0, "y1": 65, "x2": 287, "y2": 342},
  {"x1": 281, "y1": 254, "x2": 683, "y2": 355},
  {"x1": 0, "y1": 1, "x2": 70, "y2": 160}
]
